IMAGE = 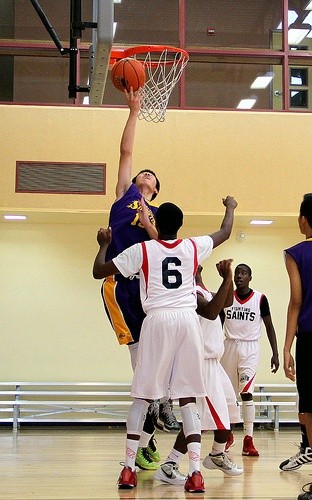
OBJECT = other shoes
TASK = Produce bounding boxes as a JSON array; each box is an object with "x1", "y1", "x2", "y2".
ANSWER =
[{"x1": 297, "y1": 482, "x2": 312, "y2": 500}]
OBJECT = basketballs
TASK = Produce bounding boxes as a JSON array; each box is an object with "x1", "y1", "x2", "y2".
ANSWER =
[{"x1": 110, "y1": 57, "x2": 146, "y2": 94}]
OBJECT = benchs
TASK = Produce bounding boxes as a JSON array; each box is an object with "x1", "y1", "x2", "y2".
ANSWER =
[{"x1": 0, "y1": 380, "x2": 300, "y2": 432}]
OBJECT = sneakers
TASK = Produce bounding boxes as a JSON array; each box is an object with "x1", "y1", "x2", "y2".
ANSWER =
[
  {"x1": 184, "y1": 470, "x2": 205, "y2": 492},
  {"x1": 152, "y1": 461, "x2": 186, "y2": 484},
  {"x1": 147, "y1": 435, "x2": 160, "y2": 461},
  {"x1": 148, "y1": 401, "x2": 164, "y2": 433},
  {"x1": 203, "y1": 452, "x2": 244, "y2": 476},
  {"x1": 242, "y1": 435, "x2": 259, "y2": 455},
  {"x1": 225, "y1": 433, "x2": 235, "y2": 451},
  {"x1": 279, "y1": 442, "x2": 312, "y2": 471},
  {"x1": 117, "y1": 462, "x2": 137, "y2": 488},
  {"x1": 135, "y1": 447, "x2": 160, "y2": 470},
  {"x1": 156, "y1": 402, "x2": 181, "y2": 432}
]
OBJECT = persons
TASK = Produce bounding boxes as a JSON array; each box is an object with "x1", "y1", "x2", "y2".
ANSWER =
[
  {"x1": 92, "y1": 195, "x2": 312, "y2": 500},
  {"x1": 102, "y1": 87, "x2": 184, "y2": 430}
]
